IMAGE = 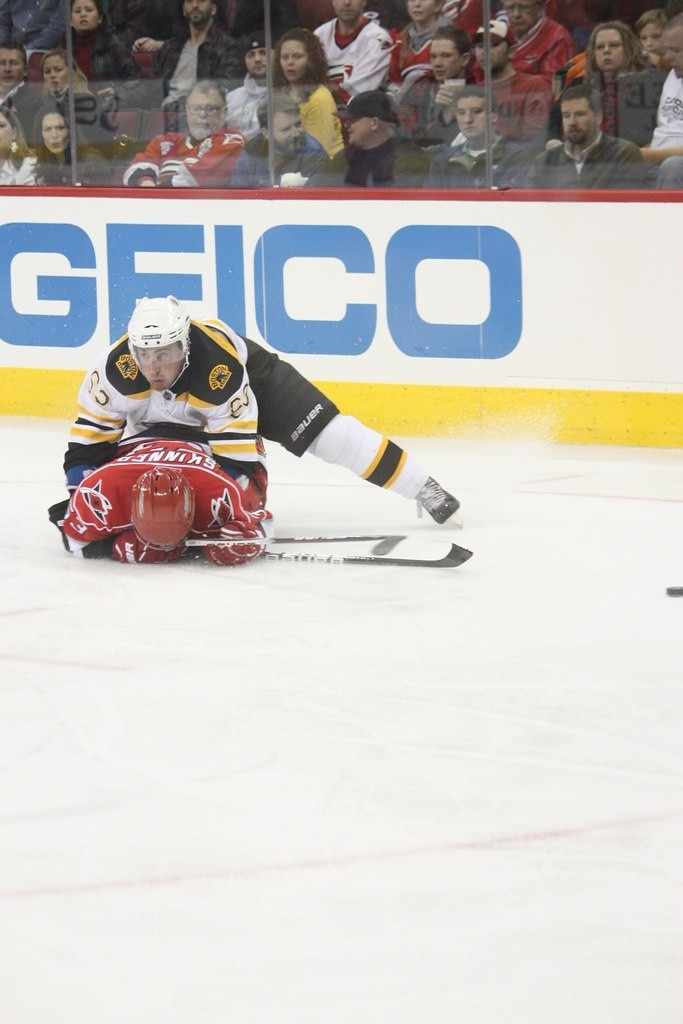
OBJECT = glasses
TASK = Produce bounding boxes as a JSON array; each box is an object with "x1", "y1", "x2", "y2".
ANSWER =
[{"x1": 187, "y1": 104, "x2": 224, "y2": 115}]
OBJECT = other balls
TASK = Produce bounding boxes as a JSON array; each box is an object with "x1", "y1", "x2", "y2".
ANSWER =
[{"x1": 666, "y1": 586, "x2": 683, "y2": 596}]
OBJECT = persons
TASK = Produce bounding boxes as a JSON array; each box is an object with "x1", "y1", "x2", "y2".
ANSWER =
[
  {"x1": 0, "y1": 0, "x2": 683, "y2": 190},
  {"x1": 62, "y1": 297, "x2": 462, "y2": 529},
  {"x1": 64, "y1": 434, "x2": 274, "y2": 565}
]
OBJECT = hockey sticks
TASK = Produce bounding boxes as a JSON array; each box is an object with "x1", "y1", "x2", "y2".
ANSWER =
[{"x1": 178, "y1": 526, "x2": 478, "y2": 567}]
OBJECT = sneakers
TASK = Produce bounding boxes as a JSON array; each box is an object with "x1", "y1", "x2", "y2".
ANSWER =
[{"x1": 414, "y1": 475, "x2": 471, "y2": 531}]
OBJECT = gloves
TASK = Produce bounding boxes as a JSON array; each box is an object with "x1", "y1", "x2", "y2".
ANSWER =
[
  {"x1": 112, "y1": 531, "x2": 186, "y2": 563},
  {"x1": 202, "y1": 521, "x2": 262, "y2": 567}
]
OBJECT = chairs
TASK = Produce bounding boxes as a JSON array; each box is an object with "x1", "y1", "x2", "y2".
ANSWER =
[{"x1": 16, "y1": 0, "x2": 391, "y2": 146}]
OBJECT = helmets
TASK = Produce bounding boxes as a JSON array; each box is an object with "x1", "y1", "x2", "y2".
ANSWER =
[
  {"x1": 130, "y1": 468, "x2": 196, "y2": 550},
  {"x1": 127, "y1": 295, "x2": 190, "y2": 368}
]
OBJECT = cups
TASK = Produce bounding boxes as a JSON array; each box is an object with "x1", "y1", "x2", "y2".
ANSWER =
[{"x1": 444, "y1": 79, "x2": 465, "y2": 117}]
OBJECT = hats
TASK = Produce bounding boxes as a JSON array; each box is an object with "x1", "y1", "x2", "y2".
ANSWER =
[
  {"x1": 331, "y1": 90, "x2": 400, "y2": 125},
  {"x1": 241, "y1": 31, "x2": 265, "y2": 56},
  {"x1": 471, "y1": 19, "x2": 514, "y2": 46}
]
